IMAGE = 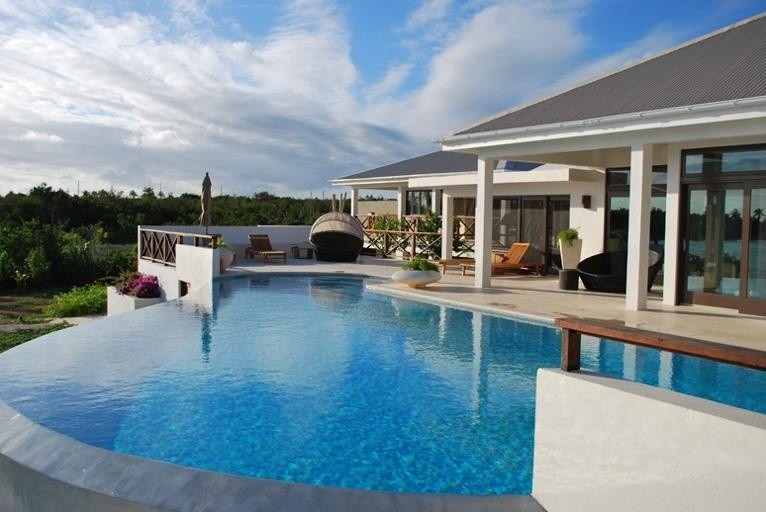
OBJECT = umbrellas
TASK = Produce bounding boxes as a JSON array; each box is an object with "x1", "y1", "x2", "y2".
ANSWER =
[
  {"x1": 199, "y1": 312, "x2": 212, "y2": 368},
  {"x1": 198, "y1": 170, "x2": 213, "y2": 235}
]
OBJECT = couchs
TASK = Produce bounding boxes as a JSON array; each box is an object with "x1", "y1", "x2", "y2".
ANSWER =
[{"x1": 576, "y1": 247, "x2": 663, "y2": 296}]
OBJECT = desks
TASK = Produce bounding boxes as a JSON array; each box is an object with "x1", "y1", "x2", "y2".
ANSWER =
[{"x1": 440, "y1": 259, "x2": 474, "y2": 274}]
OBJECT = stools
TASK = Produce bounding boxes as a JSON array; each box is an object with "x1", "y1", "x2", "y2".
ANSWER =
[{"x1": 558, "y1": 268, "x2": 580, "y2": 290}]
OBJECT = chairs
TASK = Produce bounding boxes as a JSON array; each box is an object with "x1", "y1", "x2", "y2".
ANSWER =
[
  {"x1": 244, "y1": 233, "x2": 287, "y2": 266},
  {"x1": 459, "y1": 242, "x2": 541, "y2": 277}
]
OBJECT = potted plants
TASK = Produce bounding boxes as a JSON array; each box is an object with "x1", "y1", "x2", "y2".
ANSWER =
[
  {"x1": 556, "y1": 228, "x2": 583, "y2": 270},
  {"x1": 390, "y1": 258, "x2": 442, "y2": 290},
  {"x1": 207, "y1": 233, "x2": 235, "y2": 272}
]
